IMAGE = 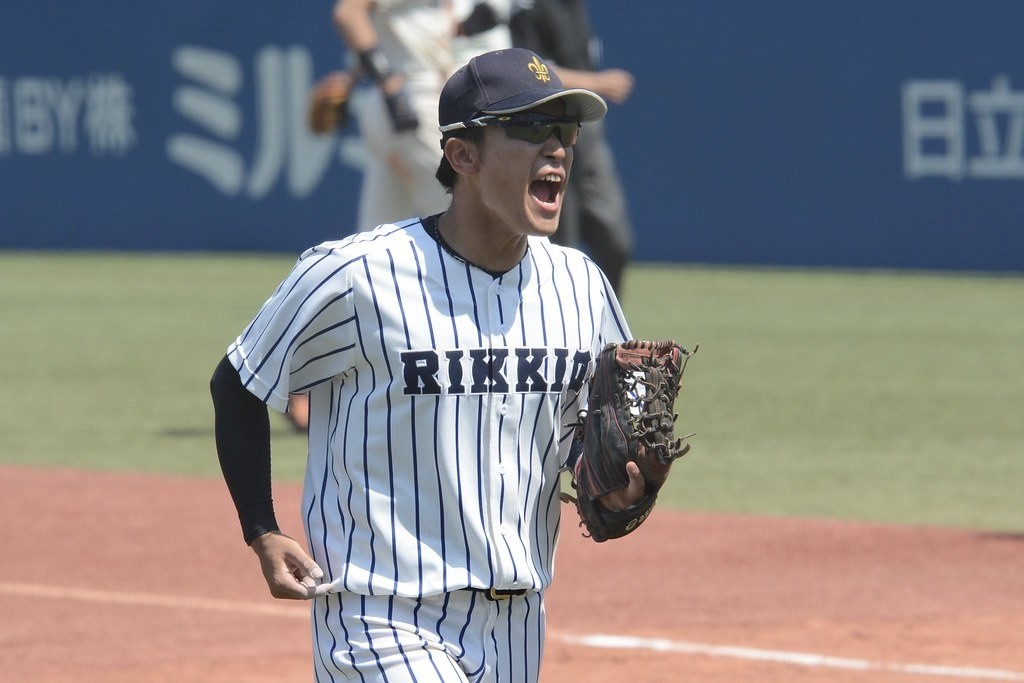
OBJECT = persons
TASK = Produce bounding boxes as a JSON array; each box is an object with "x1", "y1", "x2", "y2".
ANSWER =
[
  {"x1": 210, "y1": 48, "x2": 687, "y2": 683},
  {"x1": 282, "y1": 0, "x2": 638, "y2": 435}
]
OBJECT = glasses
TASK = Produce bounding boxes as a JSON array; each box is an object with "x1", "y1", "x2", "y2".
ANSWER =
[{"x1": 487, "y1": 109, "x2": 585, "y2": 147}]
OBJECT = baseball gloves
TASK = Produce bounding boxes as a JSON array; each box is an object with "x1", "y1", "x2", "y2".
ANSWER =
[{"x1": 557, "y1": 335, "x2": 702, "y2": 544}]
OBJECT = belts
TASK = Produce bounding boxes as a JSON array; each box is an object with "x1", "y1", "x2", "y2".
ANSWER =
[{"x1": 460, "y1": 586, "x2": 529, "y2": 601}]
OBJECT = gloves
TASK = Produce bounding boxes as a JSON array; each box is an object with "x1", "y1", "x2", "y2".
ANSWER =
[{"x1": 385, "y1": 89, "x2": 418, "y2": 133}]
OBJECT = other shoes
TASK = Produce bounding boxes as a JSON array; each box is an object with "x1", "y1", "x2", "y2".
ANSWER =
[{"x1": 284, "y1": 390, "x2": 309, "y2": 430}]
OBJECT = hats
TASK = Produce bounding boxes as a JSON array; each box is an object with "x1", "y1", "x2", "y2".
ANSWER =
[{"x1": 437, "y1": 47, "x2": 609, "y2": 149}]
